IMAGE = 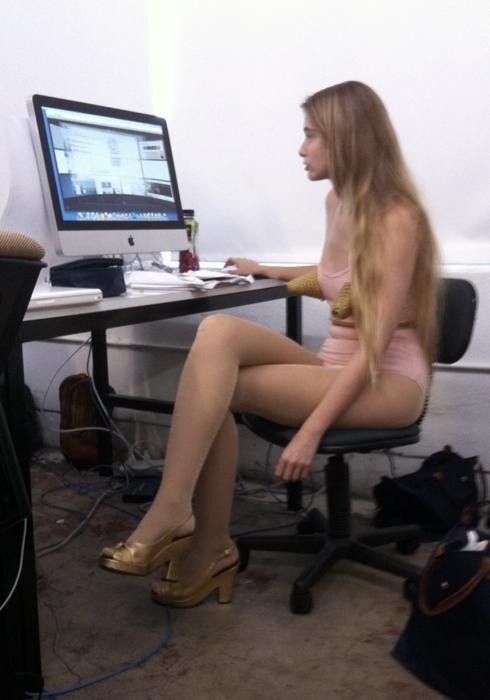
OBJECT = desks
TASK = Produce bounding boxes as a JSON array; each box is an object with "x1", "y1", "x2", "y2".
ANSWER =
[{"x1": 8, "y1": 262, "x2": 302, "y2": 696}]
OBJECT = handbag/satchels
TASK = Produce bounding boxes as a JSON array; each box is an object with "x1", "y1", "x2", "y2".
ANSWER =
[
  {"x1": 370, "y1": 446, "x2": 484, "y2": 538},
  {"x1": 388, "y1": 521, "x2": 489, "y2": 699}
]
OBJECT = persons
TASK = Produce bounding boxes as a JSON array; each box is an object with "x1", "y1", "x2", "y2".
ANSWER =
[{"x1": 100, "y1": 82, "x2": 436, "y2": 608}]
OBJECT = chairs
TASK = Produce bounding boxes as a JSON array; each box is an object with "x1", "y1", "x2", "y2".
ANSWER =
[
  {"x1": 0, "y1": 227, "x2": 48, "y2": 700},
  {"x1": 232, "y1": 277, "x2": 479, "y2": 616}
]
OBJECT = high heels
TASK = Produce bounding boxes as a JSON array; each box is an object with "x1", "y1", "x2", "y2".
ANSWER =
[
  {"x1": 97, "y1": 511, "x2": 197, "y2": 583},
  {"x1": 149, "y1": 539, "x2": 242, "y2": 609}
]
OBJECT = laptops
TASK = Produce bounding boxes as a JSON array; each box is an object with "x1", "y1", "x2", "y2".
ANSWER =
[{"x1": 26, "y1": 279, "x2": 105, "y2": 313}]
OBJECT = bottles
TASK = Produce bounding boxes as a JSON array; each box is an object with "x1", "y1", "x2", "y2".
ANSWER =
[{"x1": 179, "y1": 209, "x2": 199, "y2": 270}]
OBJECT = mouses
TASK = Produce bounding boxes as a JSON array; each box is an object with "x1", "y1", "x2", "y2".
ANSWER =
[{"x1": 220, "y1": 263, "x2": 238, "y2": 276}]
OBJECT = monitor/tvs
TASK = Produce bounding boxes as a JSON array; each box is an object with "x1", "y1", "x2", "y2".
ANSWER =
[{"x1": 21, "y1": 92, "x2": 187, "y2": 271}]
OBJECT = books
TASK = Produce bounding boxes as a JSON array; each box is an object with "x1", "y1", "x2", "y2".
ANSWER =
[{"x1": 123, "y1": 270, "x2": 255, "y2": 292}]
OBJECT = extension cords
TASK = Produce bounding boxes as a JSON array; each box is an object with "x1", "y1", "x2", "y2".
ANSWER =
[{"x1": 116, "y1": 458, "x2": 163, "y2": 475}]
